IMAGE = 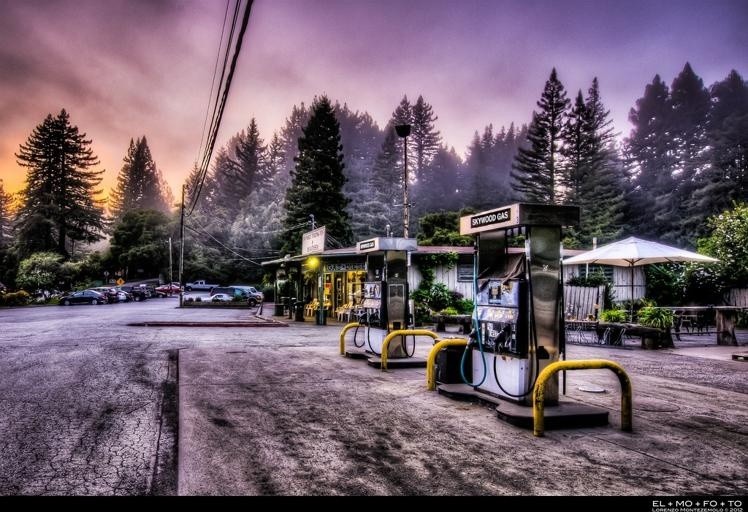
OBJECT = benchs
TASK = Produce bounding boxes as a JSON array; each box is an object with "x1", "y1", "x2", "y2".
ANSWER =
[
  {"x1": 435, "y1": 311, "x2": 471, "y2": 334},
  {"x1": 561, "y1": 321, "x2": 670, "y2": 349}
]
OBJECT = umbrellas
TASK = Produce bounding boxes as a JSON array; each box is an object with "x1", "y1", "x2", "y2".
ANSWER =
[{"x1": 559, "y1": 235, "x2": 722, "y2": 322}]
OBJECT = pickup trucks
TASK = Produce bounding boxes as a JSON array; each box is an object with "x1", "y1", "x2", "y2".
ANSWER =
[{"x1": 185, "y1": 279, "x2": 220, "y2": 292}]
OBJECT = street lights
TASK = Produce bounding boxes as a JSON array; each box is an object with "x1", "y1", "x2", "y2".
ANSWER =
[
  {"x1": 309, "y1": 213, "x2": 316, "y2": 230},
  {"x1": 394, "y1": 122, "x2": 412, "y2": 239}
]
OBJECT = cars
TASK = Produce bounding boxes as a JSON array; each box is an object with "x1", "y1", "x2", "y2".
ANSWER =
[
  {"x1": 204, "y1": 292, "x2": 234, "y2": 303},
  {"x1": 30, "y1": 281, "x2": 181, "y2": 307},
  {"x1": 0, "y1": 281, "x2": 8, "y2": 295}
]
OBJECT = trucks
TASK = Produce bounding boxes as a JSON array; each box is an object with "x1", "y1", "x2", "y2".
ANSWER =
[{"x1": 229, "y1": 285, "x2": 265, "y2": 302}]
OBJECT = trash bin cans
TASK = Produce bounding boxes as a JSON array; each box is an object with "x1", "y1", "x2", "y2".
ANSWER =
[
  {"x1": 435, "y1": 340, "x2": 472, "y2": 383},
  {"x1": 317, "y1": 309, "x2": 328, "y2": 325},
  {"x1": 275, "y1": 305, "x2": 284, "y2": 316},
  {"x1": 294, "y1": 301, "x2": 304, "y2": 322},
  {"x1": 281, "y1": 297, "x2": 290, "y2": 310}
]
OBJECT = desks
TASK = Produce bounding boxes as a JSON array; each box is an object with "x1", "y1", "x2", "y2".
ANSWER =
[{"x1": 660, "y1": 306, "x2": 748, "y2": 346}]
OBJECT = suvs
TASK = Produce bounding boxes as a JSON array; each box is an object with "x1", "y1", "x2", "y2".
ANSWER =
[{"x1": 209, "y1": 286, "x2": 261, "y2": 307}]
